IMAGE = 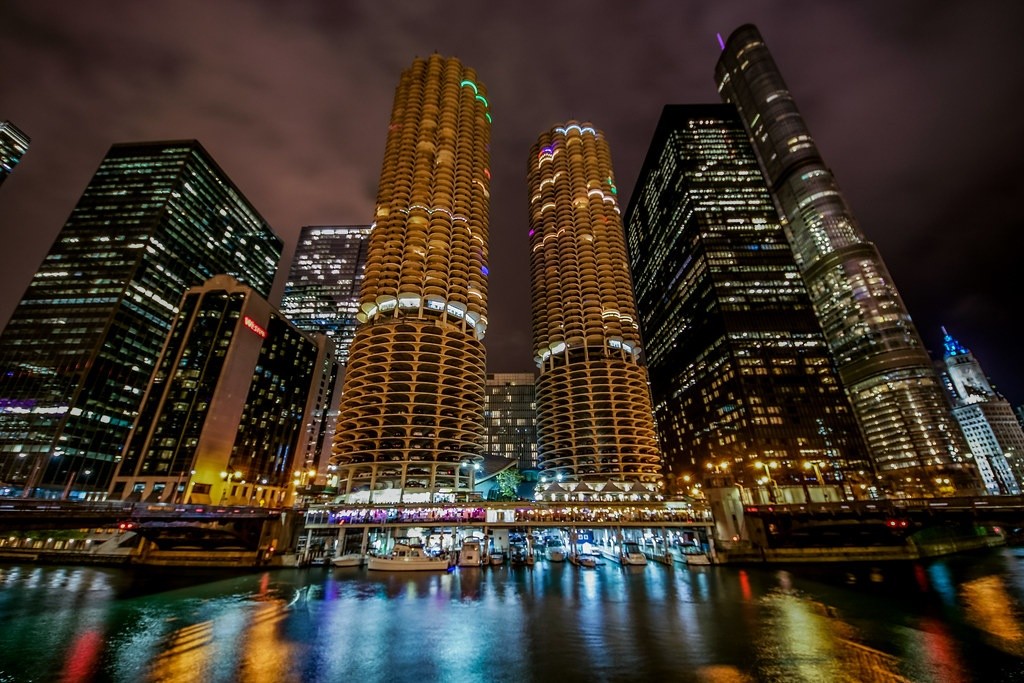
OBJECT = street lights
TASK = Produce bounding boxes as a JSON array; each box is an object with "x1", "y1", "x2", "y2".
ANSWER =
[
  {"x1": 755, "y1": 461, "x2": 784, "y2": 504},
  {"x1": 294, "y1": 471, "x2": 315, "y2": 485},
  {"x1": 707, "y1": 462, "x2": 727, "y2": 474},
  {"x1": 218, "y1": 472, "x2": 242, "y2": 506},
  {"x1": 803, "y1": 461, "x2": 830, "y2": 503}
]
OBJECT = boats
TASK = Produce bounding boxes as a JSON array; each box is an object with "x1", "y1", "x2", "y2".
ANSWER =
[
  {"x1": 332, "y1": 553, "x2": 370, "y2": 567},
  {"x1": 622, "y1": 542, "x2": 648, "y2": 565},
  {"x1": 489, "y1": 552, "x2": 503, "y2": 565},
  {"x1": 368, "y1": 536, "x2": 448, "y2": 572},
  {"x1": 545, "y1": 539, "x2": 567, "y2": 562},
  {"x1": 458, "y1": 536, "x2": 481, "y2": 567}
]
